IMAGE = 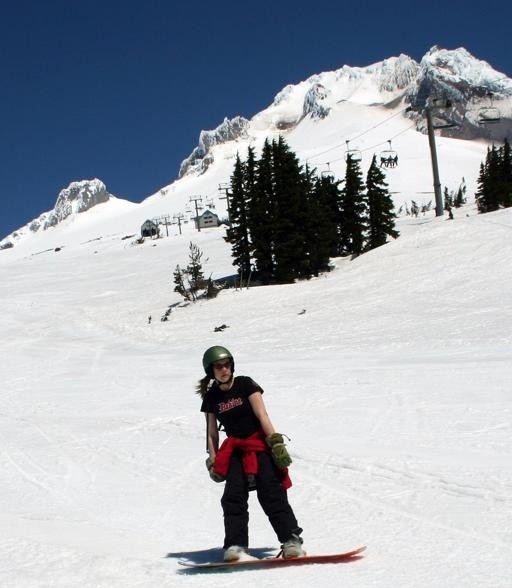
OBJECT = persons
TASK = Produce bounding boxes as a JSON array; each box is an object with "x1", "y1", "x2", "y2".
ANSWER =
[{"x1": 194, "y1": 345, "x2": 306, "y2": 563}]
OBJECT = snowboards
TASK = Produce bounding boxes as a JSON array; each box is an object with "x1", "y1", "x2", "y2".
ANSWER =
[{"x1": 177, "y1": 545, "x2": 368, "y2": 568}]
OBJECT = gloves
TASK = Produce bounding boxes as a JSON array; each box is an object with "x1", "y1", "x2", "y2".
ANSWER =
[
  {"x1": 265, "y1": 433, "x2": 292, "y2": 468},
  {"x1": 206, "y1": 458, "x2": 224, "y2": 483}
]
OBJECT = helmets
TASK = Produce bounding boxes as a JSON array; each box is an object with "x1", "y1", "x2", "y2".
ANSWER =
[{"x1": 203, "y1": 346, "x2": 234, "y2": 373}]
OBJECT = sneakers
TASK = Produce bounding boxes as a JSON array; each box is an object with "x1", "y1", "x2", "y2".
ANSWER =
[
  {"x1": 224, "y1": 545, "x2": 242, "y2": 560},
  {"x1": 282, "y1": 538, "x2": 301, "y2": 556}
]
